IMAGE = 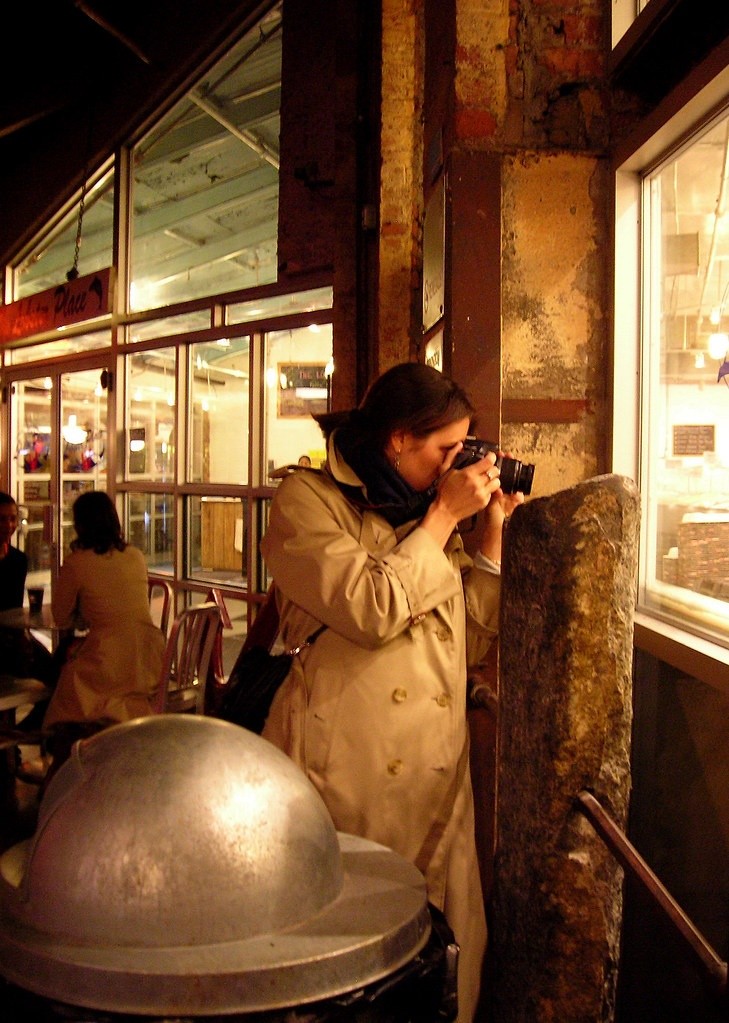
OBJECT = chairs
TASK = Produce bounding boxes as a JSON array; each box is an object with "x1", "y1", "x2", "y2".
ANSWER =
[{"x1": 146, "y1": 577, "x2": 220, "y2": 714}]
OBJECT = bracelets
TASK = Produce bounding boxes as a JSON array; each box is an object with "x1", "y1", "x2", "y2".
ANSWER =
[{"x1": 481, "y1": 551, "x2": 501, "y2": 565}]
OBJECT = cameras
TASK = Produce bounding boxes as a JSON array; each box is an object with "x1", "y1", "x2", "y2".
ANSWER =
[{"x1": 452, "y1": 435, "x2": 535, "y2": 497}]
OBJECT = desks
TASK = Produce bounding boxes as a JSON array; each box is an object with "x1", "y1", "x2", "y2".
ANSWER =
[{"x1": 0, "y1": 605, "x2": 82, "y2": 656}]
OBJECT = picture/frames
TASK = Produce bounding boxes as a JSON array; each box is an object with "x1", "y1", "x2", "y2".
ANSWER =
[{"x1": 278, "y1": 362, "x2": 328, "y2": 418}]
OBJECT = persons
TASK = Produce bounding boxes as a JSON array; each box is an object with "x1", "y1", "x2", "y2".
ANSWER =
[
  {"x1": 297, "y1": 456, "x2": 311, "y2": 468},
  {"x1": 0, "y1": 492, "x2": 167, "y2": 800},
  {"x1": 24, "y1": 434, "x2": 44, "y2": 473},
  {"x1": 260, "y1": 362, "x2": 524, "y2": 1023}
]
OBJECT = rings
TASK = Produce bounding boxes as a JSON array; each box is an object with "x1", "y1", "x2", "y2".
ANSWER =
[{"x1": 485, "y1": 471, "x2": 493, "y2": 480}]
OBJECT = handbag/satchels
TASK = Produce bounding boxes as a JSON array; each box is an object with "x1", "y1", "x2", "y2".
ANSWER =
[{"x1": 216, "y1": 645, "x2": 294, "y2": 736}]
